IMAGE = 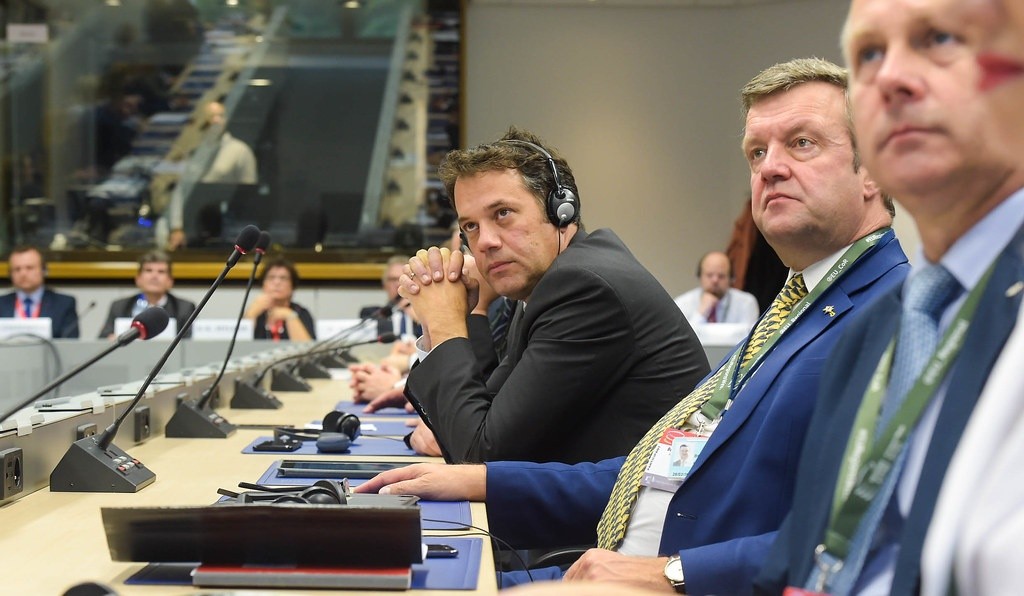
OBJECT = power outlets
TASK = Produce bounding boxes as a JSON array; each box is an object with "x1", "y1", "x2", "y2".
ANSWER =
[{"x1": 1, "y1": 383, "x2": 222, "y2": 500}]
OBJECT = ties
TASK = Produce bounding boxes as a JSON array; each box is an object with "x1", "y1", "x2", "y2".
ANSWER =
[
  {"x1": 705, "y1": 302, "x2": 718, "y2": 324},
  {"x1": 798, "y1": 265, "x2": 960, "y2": 596},
  {"x1": 396, "y1": 309, "x2": 408, "y2": 336},
  {"x1": 20, "y1": 297, "x2": 34, "y2": 318},
  {"x1": 592, "y1": 271, "x2": 809, "y2": 551}
]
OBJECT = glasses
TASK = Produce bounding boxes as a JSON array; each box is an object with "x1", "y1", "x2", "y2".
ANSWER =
[{"x1": 216, "y1": 478, "x2": 350, "y2": 498}]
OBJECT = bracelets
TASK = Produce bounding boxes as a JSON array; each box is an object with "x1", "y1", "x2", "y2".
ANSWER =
[{"x1": 286, "y1": 311, "x2": 299, "y2": 322}]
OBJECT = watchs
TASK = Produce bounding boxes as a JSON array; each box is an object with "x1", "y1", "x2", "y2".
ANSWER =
[{"x1": 662, "y1": 554, "x2": 686, "y2": 594}]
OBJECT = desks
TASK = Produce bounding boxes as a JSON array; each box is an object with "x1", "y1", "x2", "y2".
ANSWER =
[{"x1": 0, "y1": 337, "x2": 499, "y2": 596}]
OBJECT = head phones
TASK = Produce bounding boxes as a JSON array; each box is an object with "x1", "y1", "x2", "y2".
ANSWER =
[
  {"x1": 274, "y1": 409, "x2": 362, "y2": 441},
  {"x1": 504, "y1": 139, "x2": 579, "y2": 228},
  {"x1": 238, "y1": 480, "x2": 348, "y2": 504}
]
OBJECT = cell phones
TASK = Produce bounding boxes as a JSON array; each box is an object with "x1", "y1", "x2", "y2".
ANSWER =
[{"x1": 425, "y1": 544, "x2": 459, "y2": 558}]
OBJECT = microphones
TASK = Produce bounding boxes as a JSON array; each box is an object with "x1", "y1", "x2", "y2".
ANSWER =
[{"x1": 0, "y1": 224, "x2": 412, "y2": 493}]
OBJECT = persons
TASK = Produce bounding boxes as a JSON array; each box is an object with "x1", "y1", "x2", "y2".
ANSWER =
[
  {"x1": 97, "y1": 249, "x2": 196, "y2": 340},
  {"x1": 247, "y1": 257, "x2": 316, "y2": 342},
  {"x1": 673, "y1": 250, "x2": 759, "y2": 324},
  {"x1": 64, "y1": 85, "x2": 146, "y2": 174},
  {"x1": 398, "y1": 123, "x2": 714, "y2": 466},
  {"x1": 350, "y1": 0, "x2": 1024, "y2": 596},
  {"x1": 0, "y1": 242, "x2": 80, "y2": 338},
  {"x1": 673, "y1": 444, "x2": 692, "y2": 466},
  {"x1": 167, "y1": 102, "x2": 257, "y2": 254}
]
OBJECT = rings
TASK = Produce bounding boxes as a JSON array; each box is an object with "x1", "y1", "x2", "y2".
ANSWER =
[{"x1": 408, "y1": 272, "x2": 416, "y2": 280}]
